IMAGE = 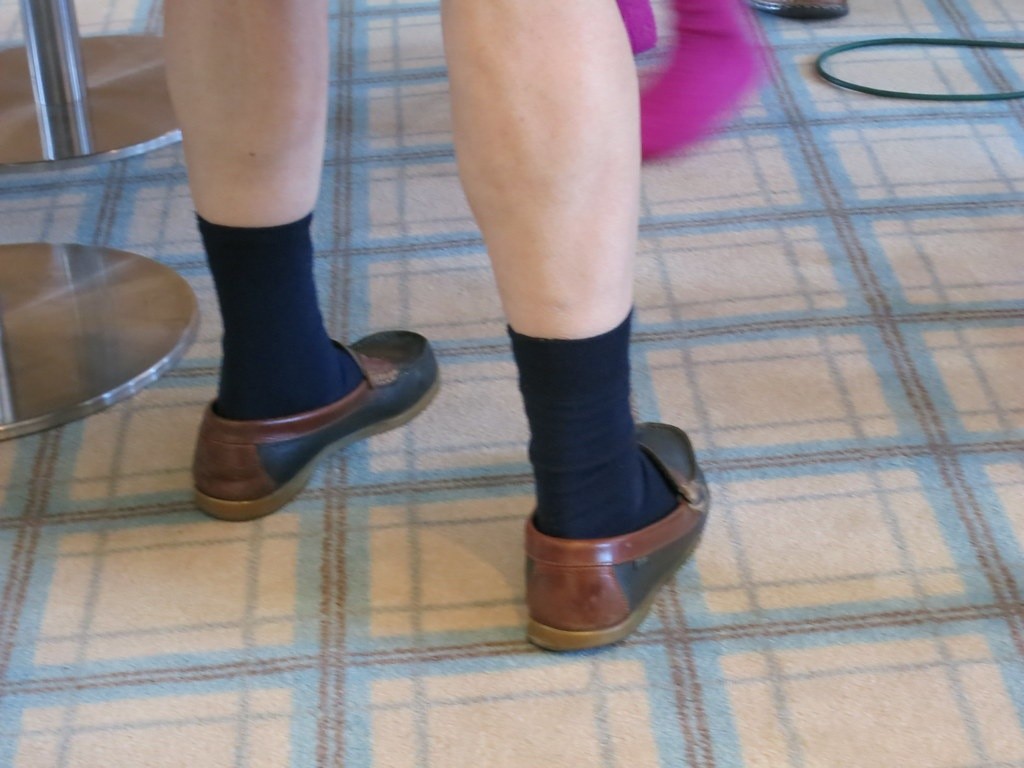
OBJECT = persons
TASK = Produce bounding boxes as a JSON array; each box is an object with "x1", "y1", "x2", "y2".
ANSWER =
[{"x1": 161, "y1": 0, "x2": 710, "y2": 649}]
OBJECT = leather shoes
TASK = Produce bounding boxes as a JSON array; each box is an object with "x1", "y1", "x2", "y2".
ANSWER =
[
  {"x1": 518, "y1": 421, "x2": 711, "y2": 653},
  {"x1": 190, "y1": 328, "x2": 440, "y2": 522}
]
